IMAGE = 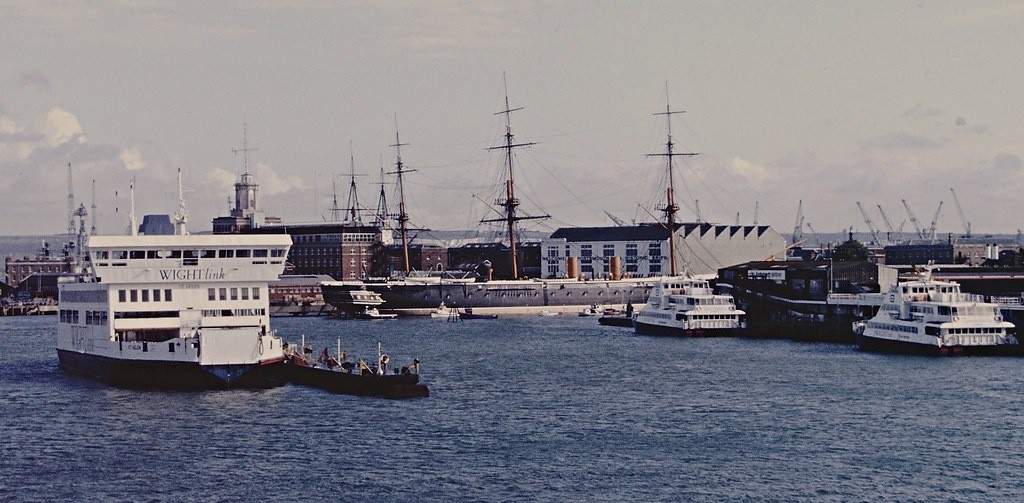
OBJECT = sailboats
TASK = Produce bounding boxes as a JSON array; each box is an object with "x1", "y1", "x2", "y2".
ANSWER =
[
  {"x1": 318, "y1": 75, "x2": 658, "y2": 318},
  {"x1": 635, "y1": 83, "x2": 746, "y2": 336}
]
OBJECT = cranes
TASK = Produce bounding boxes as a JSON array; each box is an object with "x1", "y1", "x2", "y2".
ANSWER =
[{"x1": 694, "y1": 186, "x2": 980, "y2": 249}]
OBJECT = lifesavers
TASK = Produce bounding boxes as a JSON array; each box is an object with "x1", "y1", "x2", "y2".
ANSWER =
[
  {"x1": 953, "y1": 316, "x2": 960, "y2": 323},
  {"x1": 697, "y1": 306, "x2": 702, "y2": 311},
  {"x1": 381, "y1": 353, "x2": 390, "y2": 364}
]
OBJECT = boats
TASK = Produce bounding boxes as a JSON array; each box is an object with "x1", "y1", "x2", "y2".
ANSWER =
[
  {"x1": 54, "y1": 162, "x2": 294, "y2": 391},
  {"x1": 854, "y1": 260, "x2": 1016, "y2": 356}
]
